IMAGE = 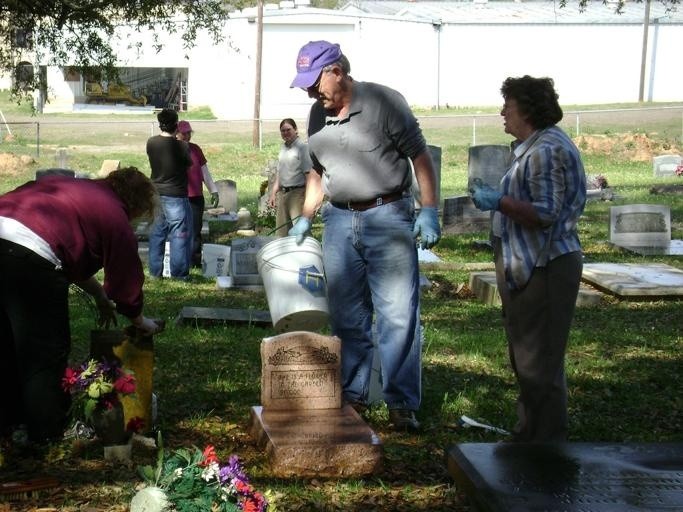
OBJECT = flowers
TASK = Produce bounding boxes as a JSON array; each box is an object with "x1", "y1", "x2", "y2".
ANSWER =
[
  {"x1": 160, "y1": 444, "x2": 281, "y2": 512},
  {"x1": 62, "y1": 360, "x2": 137, "y2": 425}
]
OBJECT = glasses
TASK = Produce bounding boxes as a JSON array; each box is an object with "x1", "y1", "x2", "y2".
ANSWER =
[{"x1": 301, "y1": 73, "x2": 331, "y2": 93}]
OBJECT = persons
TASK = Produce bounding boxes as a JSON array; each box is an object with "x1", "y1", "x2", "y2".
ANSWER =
[
  {"x1": 470, "y1": 76, "x2": 587, "y2": 443},
  {"x1": 269, "y1": 119, "x2": 315, "y2": 239},
  {"x1": 148, "y1": 108, "x2": 194, "y2": 282},
  {"x1": 287, "y1": 41, "x2": 442, "y2": 432},
  {"x1": 0, "y1": 166, "x2": 160, "y2": 457},
  {"x1": 175, "y1": 121, "x2": 219, "y2": 269}
]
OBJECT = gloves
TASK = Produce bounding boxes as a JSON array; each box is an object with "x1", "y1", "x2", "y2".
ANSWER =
[
  {"x1": 468, "y1": 185, "x2": 504, "y2": 213},
  {"x1": 210, "y1": 193, "x2": 220, "y2": 209},
  {"x1": 138, "y1": 314, "x2": 164, "y2": 339},
  {"x1": 412, "y1": 206, "x2": 441, "y2": 252},
  {"x1": 289, "y1": 216, "x2": 313, "y2": 244}
]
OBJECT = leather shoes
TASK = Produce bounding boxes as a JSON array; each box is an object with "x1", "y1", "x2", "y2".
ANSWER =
[{"x1": 389, "y1": 408, "x2": 421, "y2": 432}]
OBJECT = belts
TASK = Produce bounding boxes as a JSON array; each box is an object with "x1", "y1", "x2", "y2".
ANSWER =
[
  {"x1": 280, "y1": 185, "x2": 303, "y2": 194},
  {"x1": 328, "y1": 192, "x2": 404, "y2": 214}
]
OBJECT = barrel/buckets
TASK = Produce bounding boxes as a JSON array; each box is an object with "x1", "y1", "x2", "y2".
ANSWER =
[
  {"x1": 467, "y1": 145, "x2": 511, "y2": 196},
  {"x1": 162, "y1": 240, "x2": 171, "y2": 278},
  {"x1": 200, "y1": 222, "x2": 211, "y2": 237},
  {"x1": 215, "y1": 180, "x2": 238, "y2": 212},
  {"x1": 232, "y1": 237, "x2": 276, "y2": 292},
  {"x1": 408, "y1": 145, "x2": 441, "y2": 213},
  {"x1": 89, "y1": 324, "x2": 155, "y2": 435},
  {"x1": 35, "y1": 167, "x2": 75, "y2": 181},
  {"x1": 199, "y1": 243, "x2": 232, "y2": 281},
  {"x1": 253, "y1": 234, "x2": 331, "y2": 331}
]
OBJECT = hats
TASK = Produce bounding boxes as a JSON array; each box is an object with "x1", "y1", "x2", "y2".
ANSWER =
[
  {"x1": 295, "y1": 40, "x2": 341, "y2": 91},
  {"x1": 177, "y1": 119, "x2": 194, "y2": 134}
]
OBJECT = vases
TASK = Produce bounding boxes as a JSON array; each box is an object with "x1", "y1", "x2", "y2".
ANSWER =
[
  {"x1": 92, "y1": 403, "x2": 124, "y2": 444},
  {"x1": 131, "y1": 487, "x2": 172, "y2": 512}
]
